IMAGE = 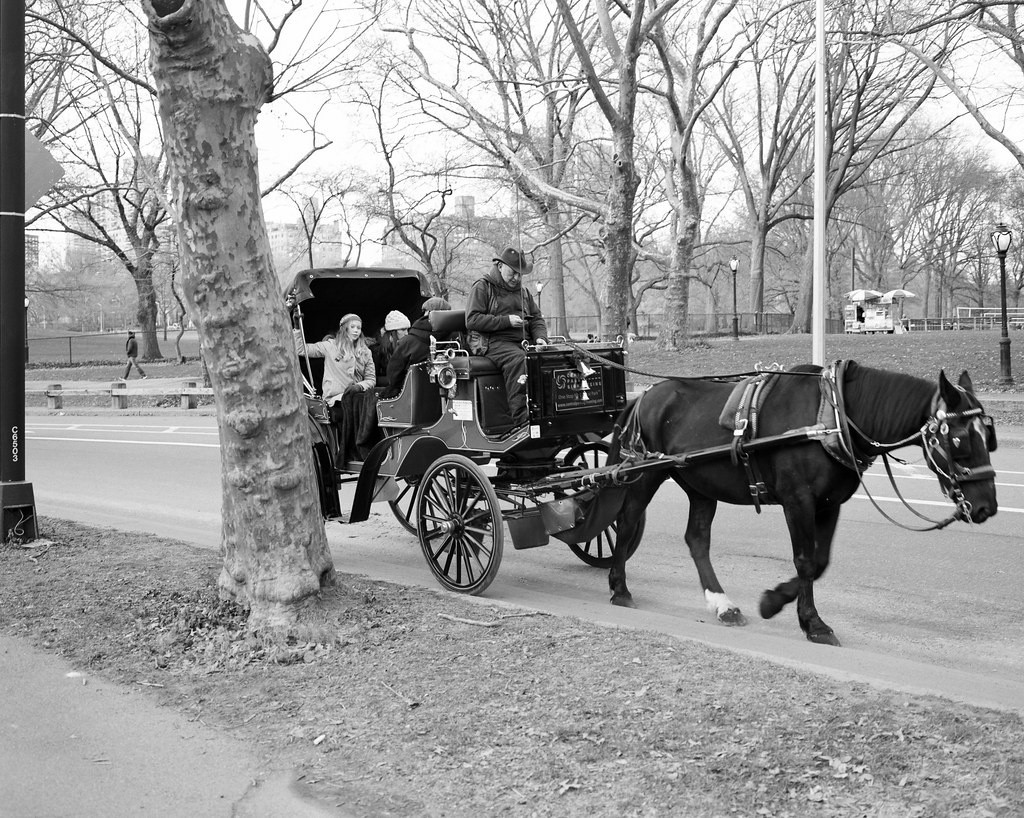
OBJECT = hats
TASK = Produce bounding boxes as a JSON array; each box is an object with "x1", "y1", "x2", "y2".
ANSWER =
[
  {"x1": 385, "y1": 310, "x2": 411, "y2": 331},
  {"x1": 339, "y1": 314, "x2": 362, "y2": 326},
  {"x1": 422, "y1": 297, "x2": 451, "y2": 312},
  {"x1": 492, "y1": 248, "x2": 533, "y2": 274}
]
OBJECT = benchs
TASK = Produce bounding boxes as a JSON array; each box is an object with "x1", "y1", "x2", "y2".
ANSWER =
[
  {"x1": 429, "y1": 309, "x2": 514, "y2": 432},
  {"x1": 377, "y1": 362, "x2": 436, "y2": 429}
]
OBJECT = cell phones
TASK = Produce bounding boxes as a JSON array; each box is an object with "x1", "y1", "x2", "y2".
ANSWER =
[{"x1": 523, "y1": 314, "x2": 534, "y2": 321}]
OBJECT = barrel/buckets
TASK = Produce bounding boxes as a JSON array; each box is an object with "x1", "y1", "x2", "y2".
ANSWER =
[
  {"x1": 508, "y1": 510, "x2": 549, "y2": 549},
  {"x1": 536, "y1": 494, "x2": 575, "y2": 533},
  {"x1": 444, "y1": 507, "x2": 486, "y2": 557}
]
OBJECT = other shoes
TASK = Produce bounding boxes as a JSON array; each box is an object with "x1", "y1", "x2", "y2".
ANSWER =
[
  {"x1": 119, "y1": 376, "x2": 127, "y2": 381},
  {"x1": 139, "y1": 375, "x2": 147, "y2": 380}
]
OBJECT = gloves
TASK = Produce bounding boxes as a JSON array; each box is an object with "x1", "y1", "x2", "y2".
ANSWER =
[
  {"x1": 292, "y1": 308, "x2": 304, "y2": 329},
  {"x1": 343, "y1": 385, "x2": 362, "y2": 398}
]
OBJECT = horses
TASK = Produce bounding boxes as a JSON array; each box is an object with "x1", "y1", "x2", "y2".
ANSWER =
[{"x1": 606, "y1": 350, "x2": 999, "y2": 645}]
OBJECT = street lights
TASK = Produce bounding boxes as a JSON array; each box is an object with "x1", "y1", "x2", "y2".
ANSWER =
[
  {"x1": 25, "y1": 295, "x2": 31, "y2": 364},
  {"x1": 989, "y1": 222, "x2": 1016, "y2": 387},
  {"x1": 535, "y1": 280, "x2": 543, "y2": 310},
  {"x1": 728, "y1": 254, "x2": 740, "y2": 340}
]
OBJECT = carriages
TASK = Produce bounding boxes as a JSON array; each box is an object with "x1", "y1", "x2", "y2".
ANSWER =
[{"x1": 284, "y1": 268, "x2": 1001, "y2": 649}]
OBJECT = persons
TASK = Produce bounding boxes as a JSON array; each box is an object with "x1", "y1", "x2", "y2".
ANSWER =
[
  {"x1": 119, "y1": 331, "x2": 147, "y2": 380},
  {"x1": 386, "y1": 296, "x2": 452, "y2": 386},
  {"x1": 465, "y1": 248, "x2": 550, "y2": 426},
  {"x1": 901, "y1": 314, "x2": 908, "y2": 320},
  {"x1": 367, "y1": 310, "x2": 411, "y2": 386},
  {"x1": 587, "y1": 334, "x2": 597, "y2": 343},
  {"x1": 293, "y1": 308, "x2": 376, "y2": 468},
  {"x1": 854, "y1": 303, "x2": 865, "y2": 323}
]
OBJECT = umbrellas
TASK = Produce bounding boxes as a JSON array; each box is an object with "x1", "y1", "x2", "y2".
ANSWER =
[
  {"x1": 883, "y1": 289, "x2": 916, "y2": 298},
  {"x1": 846, "y1": 289, "x2": 883, "y2": 301}
]
